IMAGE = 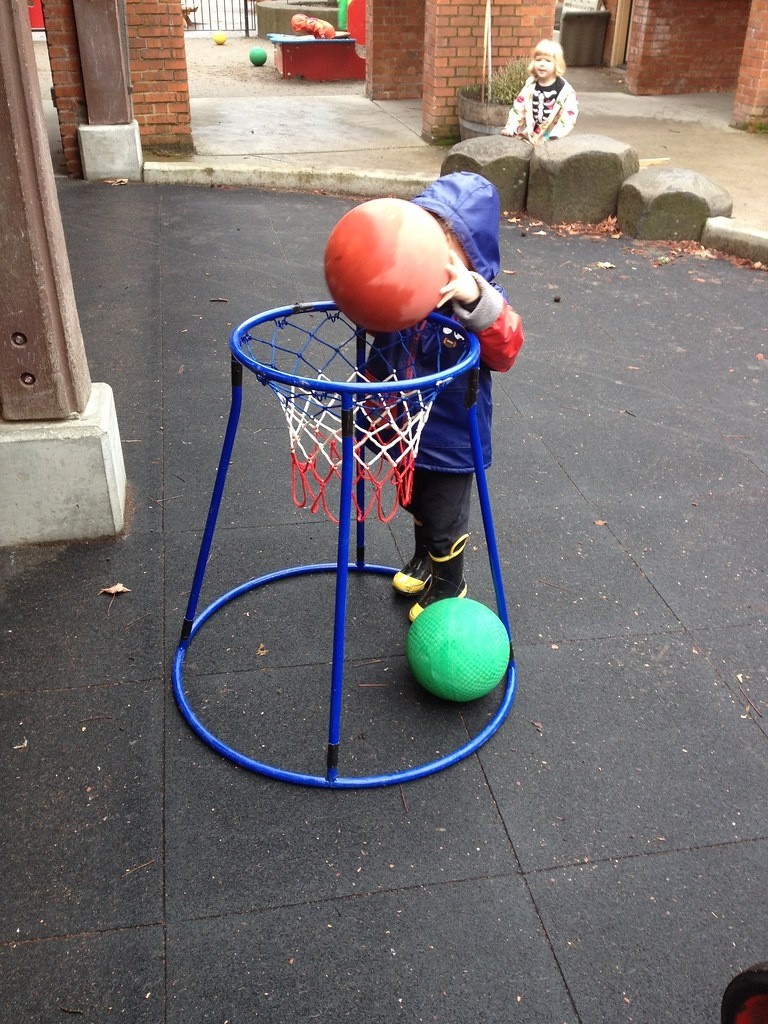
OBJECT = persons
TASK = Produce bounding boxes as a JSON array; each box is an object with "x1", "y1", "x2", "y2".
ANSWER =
[
  {"x1": 290, "y1": 14, "x2": 336, "y2": 39},
  {"x1": 355, "y1": 171, "x2": 524, "y2": 624},
  {"x1": 500, "y1": 38, "x2": 579, "y2": 147}
]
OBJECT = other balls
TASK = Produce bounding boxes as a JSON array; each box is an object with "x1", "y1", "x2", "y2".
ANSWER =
[
  {"x1": 323, "y1": 197, "x2": 451, "y2": 331},
  {"x1": 212, "y1": 32, "x2": 227, "y2": 45},
  {"x1": 247, "y1": 47, "x2": 267, "y2": 67},
  {"x1": 405, "y1": 595, "x2": 511, "y2": 704}
]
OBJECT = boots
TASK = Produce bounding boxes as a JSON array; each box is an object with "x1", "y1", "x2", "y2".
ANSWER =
[
  {"x1": 392, "y1": 515, "x2": 432, "y2": 596},
  {"x1": 409, "y1": 534, "x2": 470, "y2": 623}
]
